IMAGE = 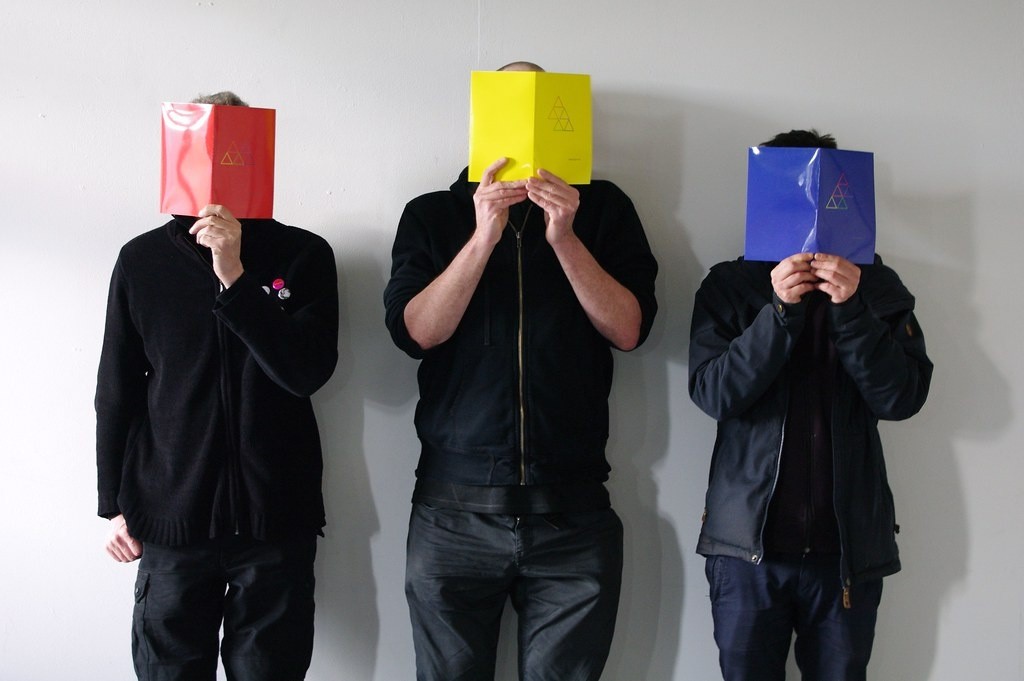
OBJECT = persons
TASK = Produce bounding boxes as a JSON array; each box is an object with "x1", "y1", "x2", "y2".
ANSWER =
[
  {"x1": 384, "y1": 60, "x2": 659, "y2": 681},
  {"x1": 688, "y1": 128, "x2": 934, "y2": 681}
]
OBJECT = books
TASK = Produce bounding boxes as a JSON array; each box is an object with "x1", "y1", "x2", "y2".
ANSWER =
[
  {"x1": 154, "y1": 103, "x2": 276, "y2": 221},
  {"x1": 94, "y1": 91, "x2": 341, "y2": 681},
  {"x1": 743, "y1": 145, "x2": 876, "y2": 266},
  {"x1": 466, "y1": 70, "x2": 592, "y2": 186}
]
